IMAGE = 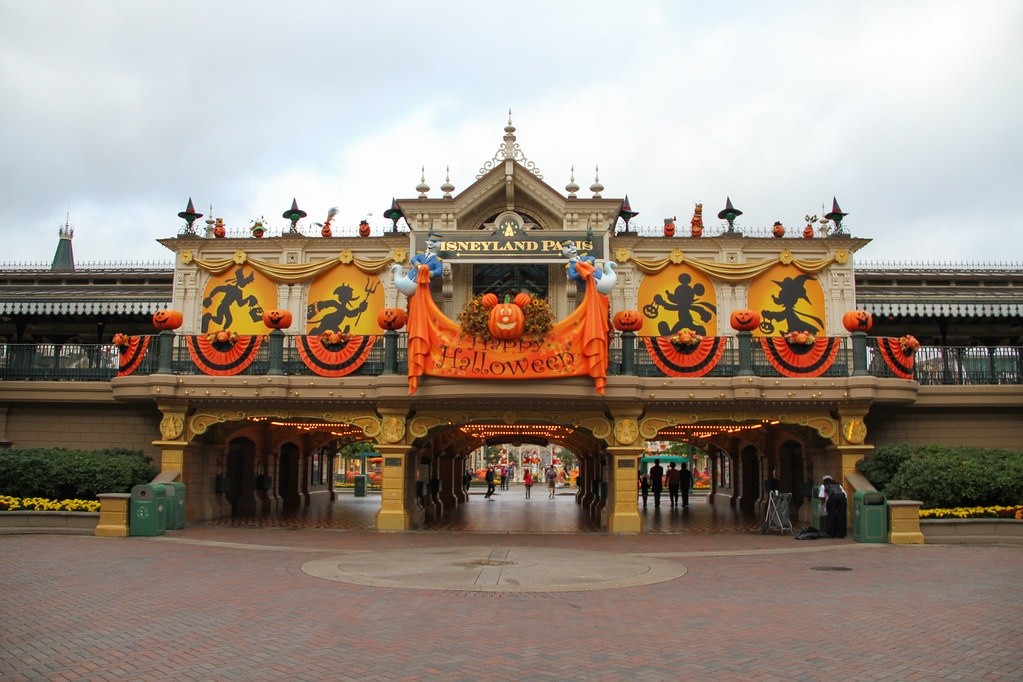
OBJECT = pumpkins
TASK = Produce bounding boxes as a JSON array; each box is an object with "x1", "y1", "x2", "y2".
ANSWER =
[{"x1": 481, "y1": 292, "x2": 531, "y2": 309}]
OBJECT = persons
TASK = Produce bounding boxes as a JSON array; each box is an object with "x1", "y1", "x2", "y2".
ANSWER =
[
  {"x1": 640, "y1": 476, "x2": 650, "y2": 506},
  {"x1": 679, "y1": 463, "x2": 694, "y2": 506},
  {"x1": 692, "y1": 466, "x2": 698, "y2": 476},
  {"x1": 523, "y1": 469, "x2": 532, "y2": 499},
  {"x1": 465, "y1": 469, "x2": 473, "y2": 491},
  {"x1": 546, "y1": 464, "x2": 557, "y2": 499},
  {"x1": 484, "y1": 465, "x2": 496, "y2": 498},
  {"x1": 703, "y1": 467, "x2": 707, "y2": 474},
  {"x1": 563, "y1": 464, "x2": 567, "y2": 476},
  {"x1": 500, "y1": 463, "x2": 508, "y2": 491},
  {"x1": 665, "y1": 462, "x2": 680, "y2": 507},
  {"x1": 649, "y1": 459, "x2": 663, "y2": 508},
  {"x1": 638, "y1": 470, "x2": 640, "y2": 503},
  {"x1": 818, "y1": 474, "x2": 847, "y2": 539}
]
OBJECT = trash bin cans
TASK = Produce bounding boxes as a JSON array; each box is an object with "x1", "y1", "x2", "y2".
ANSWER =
[
  {"x1": 852, "y1": 490, "x2": 887, "y2": 543},
  {"x1": 160, "y1": 481, "x2": 186, "y2": 530},
  {"x1": 355, "y1": 475, "x2": 367, "y2": 497},
  {"x1": 129, "y1": 484, "x2": 167, "y2": 536},
  {"x1": 811, "y1": 486, "x2": 825, "y2": 530}
]
